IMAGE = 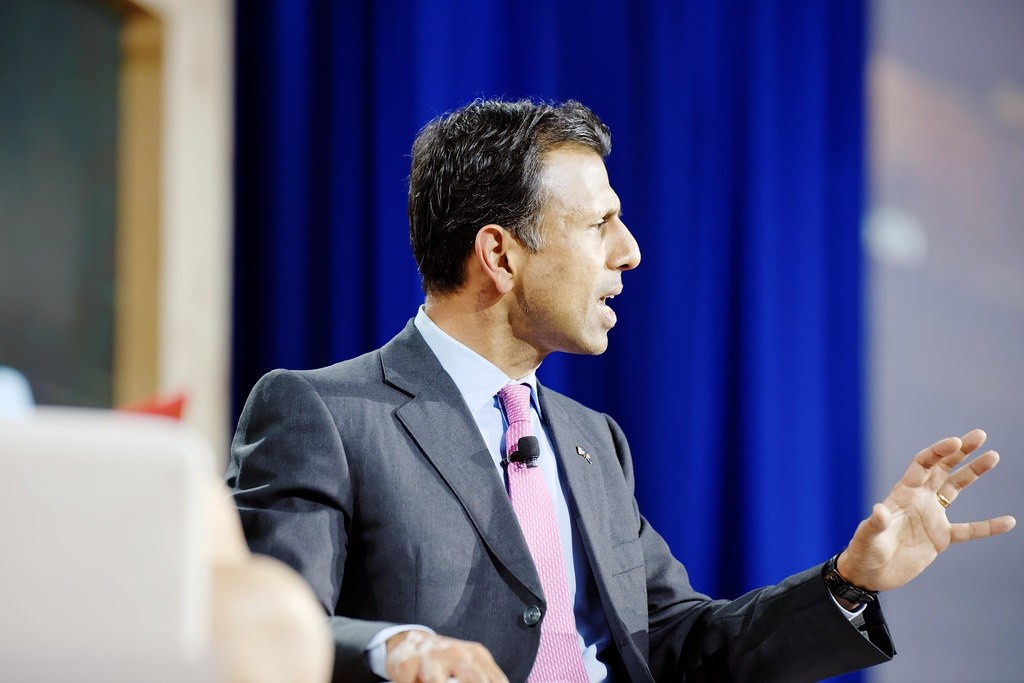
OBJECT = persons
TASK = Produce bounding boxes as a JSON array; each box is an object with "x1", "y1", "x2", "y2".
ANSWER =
[{"x1": 223, "y1": 99, "x2": 1018, "y2": 682}]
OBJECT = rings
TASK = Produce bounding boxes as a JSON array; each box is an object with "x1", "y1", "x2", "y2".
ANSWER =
[{"x1": 937, "y1": 493, "x2": 951, "y2": 508}]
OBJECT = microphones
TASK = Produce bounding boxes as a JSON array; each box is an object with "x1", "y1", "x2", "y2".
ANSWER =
[{"x1": 510, "y1": 435, "x2": 540, "y2": 468}]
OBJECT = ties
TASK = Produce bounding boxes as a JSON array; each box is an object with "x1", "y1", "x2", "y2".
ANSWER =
[{"x1": 496, "y1": 385, "x2": 591, "y2": 683}]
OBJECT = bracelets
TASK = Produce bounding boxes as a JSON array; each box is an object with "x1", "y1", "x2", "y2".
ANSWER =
[{"x1": 821, "y1": 553, "x2": 878, "y2": 606}]
